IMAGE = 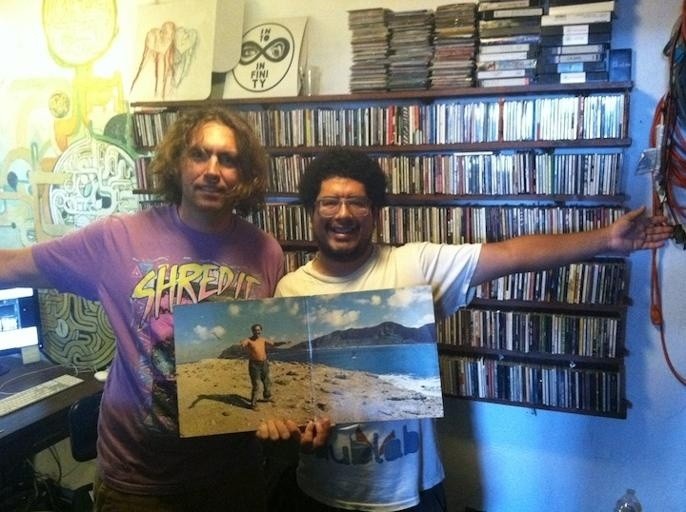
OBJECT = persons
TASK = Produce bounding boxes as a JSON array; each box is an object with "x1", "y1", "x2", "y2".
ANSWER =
[
  {"x1": 273, "y1": 144, "x2": 676, "y2": 511},
  {"x1": 240, "y1": 324, "x2": 292, "y2": 409},
  {"x1": 0, "y1": 108, "x2": 289, "y2": 512}
]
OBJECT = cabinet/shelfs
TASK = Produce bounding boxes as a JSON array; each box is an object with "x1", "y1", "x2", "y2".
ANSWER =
[{"x1": 128, "y1": 79, "x2": 631, "y2": 420}]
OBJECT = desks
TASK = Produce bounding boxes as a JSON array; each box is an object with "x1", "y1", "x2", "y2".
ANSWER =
[{"x1": 0, "y1": 356, "x2": 103, "y2": 440}]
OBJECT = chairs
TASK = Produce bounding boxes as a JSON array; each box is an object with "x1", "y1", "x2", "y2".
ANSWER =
[{"x1": 28, "y1": 394, "x2": 103, "y2": 511}]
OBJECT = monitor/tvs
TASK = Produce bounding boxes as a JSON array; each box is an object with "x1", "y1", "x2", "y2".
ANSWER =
[{"x1": 0, "y1": 286, "x2": 43, "y2": 376}]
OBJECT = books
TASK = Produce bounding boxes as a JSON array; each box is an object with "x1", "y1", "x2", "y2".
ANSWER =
[
  {"x1": 435, "y1": 305, "x2": 618, "y2": 358},
  {"x1": 376, "y1": 153, "x2": 625, "y2": 195},
  {"x1": 130, "y1": 111, "x2": 182, "y2": 211},
  {"x1": 283, "y1": 250, "x2": 313, "y2": 270},
  {"x1": 438, "y1": 356, "x2": 621, "y2": 417},
  {"x1": 348, "y1": 2, "x2": 633, "y2": 93},
  {"x1": 249, "y1": 95, "x2": 626, "y2": 146},
  {"x1": 232, "y1": 202, "x2": 316, "y2": 241},
  {"x1": 475, "y1": 262, "x2": 624, "y2": 306},
  {"x1": 273, "y1": 156, "x2": 319, "y2": 193},
  {"x1": 371, "y1": 207, "x2": 626, "y2": 245}
]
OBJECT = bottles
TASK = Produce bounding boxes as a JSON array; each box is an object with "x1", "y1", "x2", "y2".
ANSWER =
[{"x1": 613, "y1": 487, "x2": 641, "y2": 512}]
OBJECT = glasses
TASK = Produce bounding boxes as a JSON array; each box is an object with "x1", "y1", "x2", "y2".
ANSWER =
[{"x1": 317, "y1": 196, "x2": 370, "y2": 218}]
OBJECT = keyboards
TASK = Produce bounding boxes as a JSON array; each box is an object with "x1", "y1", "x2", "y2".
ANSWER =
[{"x1": 0, "y1": 374, "x2": 84, "y2": 416}]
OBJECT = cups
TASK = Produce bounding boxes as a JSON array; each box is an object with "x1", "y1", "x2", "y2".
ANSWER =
[{"x1": 301, "y1": 66, "x2": 322, "y2": 97}]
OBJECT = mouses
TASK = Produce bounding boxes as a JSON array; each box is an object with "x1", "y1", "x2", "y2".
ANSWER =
[{"x1": 94, "y1": 371, "x2": 108, "y2": 382}]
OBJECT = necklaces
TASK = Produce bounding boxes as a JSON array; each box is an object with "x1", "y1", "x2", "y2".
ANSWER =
[{"x1": 314, "y1": 251, "x2": 366, "y2": 277}]
